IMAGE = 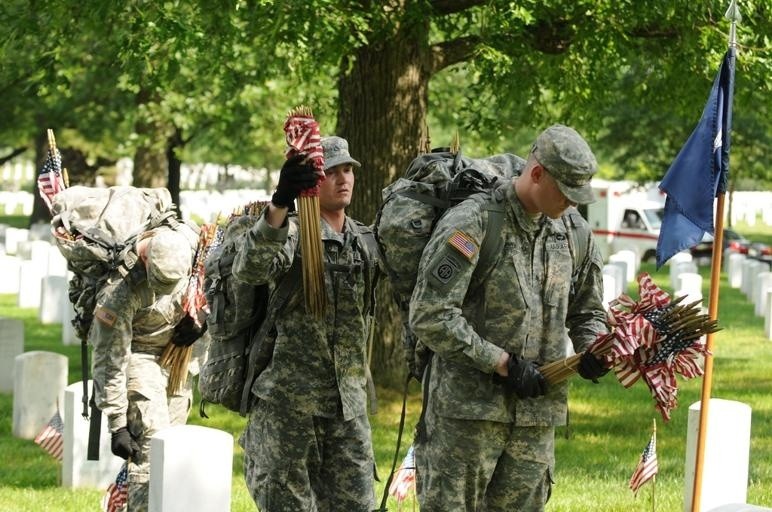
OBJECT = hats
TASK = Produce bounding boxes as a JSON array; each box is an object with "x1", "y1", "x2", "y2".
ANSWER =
[
  {"x1": 111, "y1": 429, "x2": 140, "y2": 458},
  {"x1": 320, "y1": 136, "x2": 363, "y2": 170},
  {"x1": 531, "y1": 122, "x2": 597, "y2": 205},
  {"x1": 146, "y1": 230, "x2": 191, "y2": 295}
]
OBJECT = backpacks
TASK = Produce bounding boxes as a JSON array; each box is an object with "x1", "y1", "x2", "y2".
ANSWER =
[
  {"x1": 50, "y1": 185, "x2": 200, "y2": 342},
  {"x1": 373, "y1": 146, "x2": 589, "y2": 382},
  {"x1": 197, "y1": 213, "x2": 375, "y2": 417}
]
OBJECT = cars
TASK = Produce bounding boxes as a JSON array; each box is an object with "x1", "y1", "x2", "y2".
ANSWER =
[{"x1": 723, "y1": 226, "x2": 771, "y2": 269}]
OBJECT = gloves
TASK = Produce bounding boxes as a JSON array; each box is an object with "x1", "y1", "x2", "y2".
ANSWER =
[
  {"x1": 272, "y1": 154, "x2": 321, "y2": 207},
  {"x1": 502, "y1": 351, "x2": 547, "y2": 400},
  {"x1": 578, "y1": 353, "x2": 609, "y2": 383},
  {"x1": 171, "y1": 314, "x2": 207, "y2": 347}
]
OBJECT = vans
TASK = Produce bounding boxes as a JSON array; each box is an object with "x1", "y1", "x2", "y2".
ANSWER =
[{"x1": 582, "y1": 180, "x2": 715, "y2": 266}]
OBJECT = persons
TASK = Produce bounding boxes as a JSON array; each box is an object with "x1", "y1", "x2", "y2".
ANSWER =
[
  {"x1": 91, "y1": 219, "x2": 211, "y2": 512},
  {"x1": 239, "y1": 136, "x2": 391, "y2": 512},
  {"x1": 408, "y1": 122, "x2": 612, "y2": 511}
]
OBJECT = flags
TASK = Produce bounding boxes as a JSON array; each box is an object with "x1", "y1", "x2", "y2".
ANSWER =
[
  {"x1": 390, "y1": 446, "x2": 416, "y2": 501},
  {"x1": 655, "y1": 47, "x2": 735, "y2": 270},
  {"x1": 592, "y1": 270, "x2": 713, "y2": 423},
  {"x1": 629, "y1": 430, "x2": 658, "y2": 499},
  {"x1": 38, "y1": 149, "x2": 66, "y2": 214},
  {"x1": 34, "y1": 411, "x2": 64, "y2": 462},
  {"x1": 105, "y1": 461, "x2": 128, "y2": 512}
]
